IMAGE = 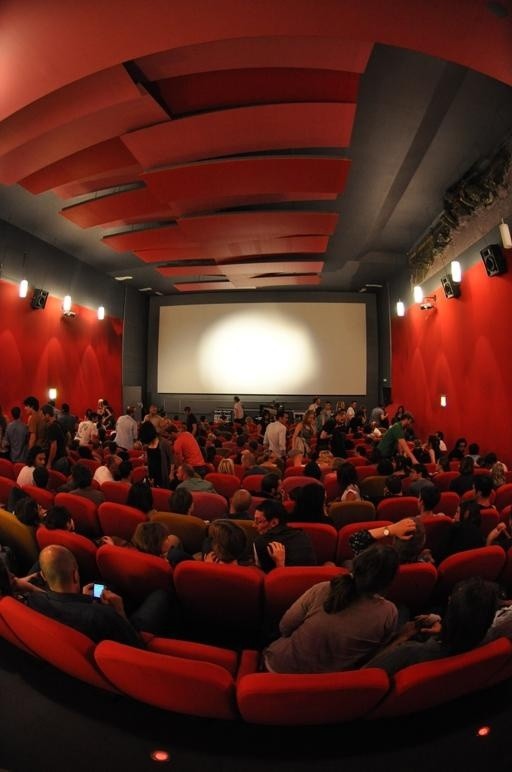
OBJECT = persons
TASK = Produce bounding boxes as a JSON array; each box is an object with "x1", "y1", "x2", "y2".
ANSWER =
[{"x1": 0, "y1": 395, "x2": 512, "y2": 674}]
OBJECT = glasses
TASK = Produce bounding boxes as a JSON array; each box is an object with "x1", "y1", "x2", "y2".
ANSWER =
[{"x1": 254, "y1": 519, "x2": 267, "y2": 524}]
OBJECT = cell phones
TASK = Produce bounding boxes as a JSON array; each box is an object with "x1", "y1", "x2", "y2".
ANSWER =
[{"x1": 92, "y1": 584, "x2": 105, "y2": 599}]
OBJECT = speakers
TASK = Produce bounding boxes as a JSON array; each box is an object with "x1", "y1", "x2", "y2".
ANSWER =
[
  {"x1": 441, "y1": 274, "x2": 458, "y2": 300},
  {"x1": 31, "y1": 288, "x2": 49, "y2": 309},
  {"x1": 479, "y1": 243, "x2": 507, "y2": 277}
]
